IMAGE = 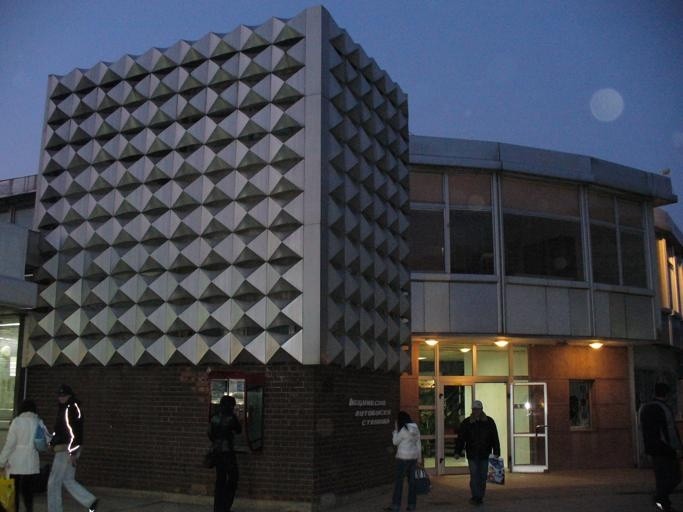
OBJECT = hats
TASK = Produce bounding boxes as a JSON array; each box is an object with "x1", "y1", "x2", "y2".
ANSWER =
[
  {"x1": 471, "y1": 400, "x2": 483, "y2": 409},
  {"x1": 52, "y1": 384, "x2": 77, "y2": 397}
]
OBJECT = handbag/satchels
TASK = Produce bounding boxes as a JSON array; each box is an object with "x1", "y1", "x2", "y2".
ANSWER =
[
  {"x1": 486, "y1": 455, "x2": 505, "y2": 485},
  {"x1": 415, "y1": 477, "x2": 430, "y2": 496},
  {"x1": 203, "y1": 449, "x2": 216, "y2": 469},
  {"x1": 34, "y1": 423, "x2": 55, "y2": 456},
  {"x1": 1, "y1": 473, "x2": 16, "y2": 511}
]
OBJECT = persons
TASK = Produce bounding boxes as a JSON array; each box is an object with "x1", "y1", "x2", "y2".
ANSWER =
[
  {"x1": 0, "y1": 400, "x2": 51, "y2": 512},
  {"x1": 382, "y1": 411, "x2": 422, "y2": 512},
  {"x1": 639, "y1": 381, "x2": 683, "y2": 512},
  {"x1": 453, "y1": 400, "x2": 501, "y2": 505},
  {"x1": 207, "y1": 395, "x2": 243, "y2": 512},
  {"x1": 46, "y1": 384, "x2": 100, "y2": 512}
]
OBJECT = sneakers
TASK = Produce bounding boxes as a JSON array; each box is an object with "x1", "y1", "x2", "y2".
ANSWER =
[
  {"x1": 469, "y1": 495, "x2": 483, "y2": 505},
  {"x1": 383, "y1": 505, "x2": 415, "y2": 511},
  {"x1": 88, "y1": 498, "x2": 101, "y2": 511}
]
